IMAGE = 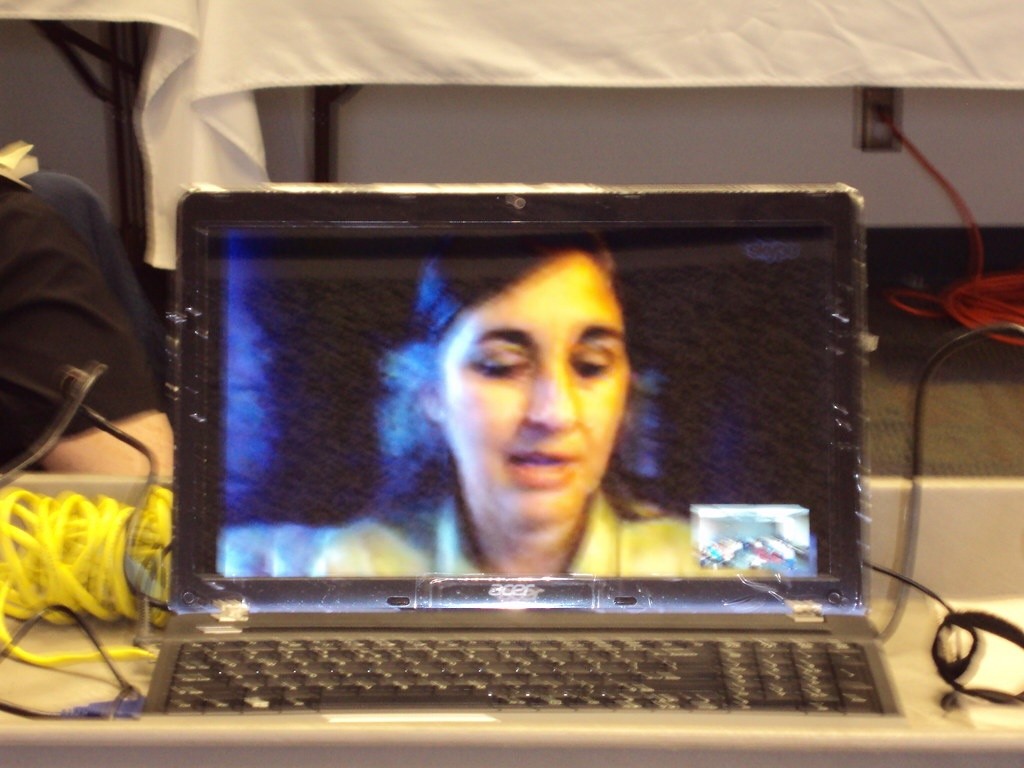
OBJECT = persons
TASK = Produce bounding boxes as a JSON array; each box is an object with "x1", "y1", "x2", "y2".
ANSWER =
[
  {"x1": 310, "y1": 234, "x2": 728, "y2": 580},
  {"x1": 0, "y1": 175, "x2": 176, "y2": 489}
]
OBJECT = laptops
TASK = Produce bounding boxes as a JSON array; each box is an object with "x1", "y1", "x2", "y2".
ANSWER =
[{"x1": 144, "y1": 182, "x2": 901, "y2": 720}]
